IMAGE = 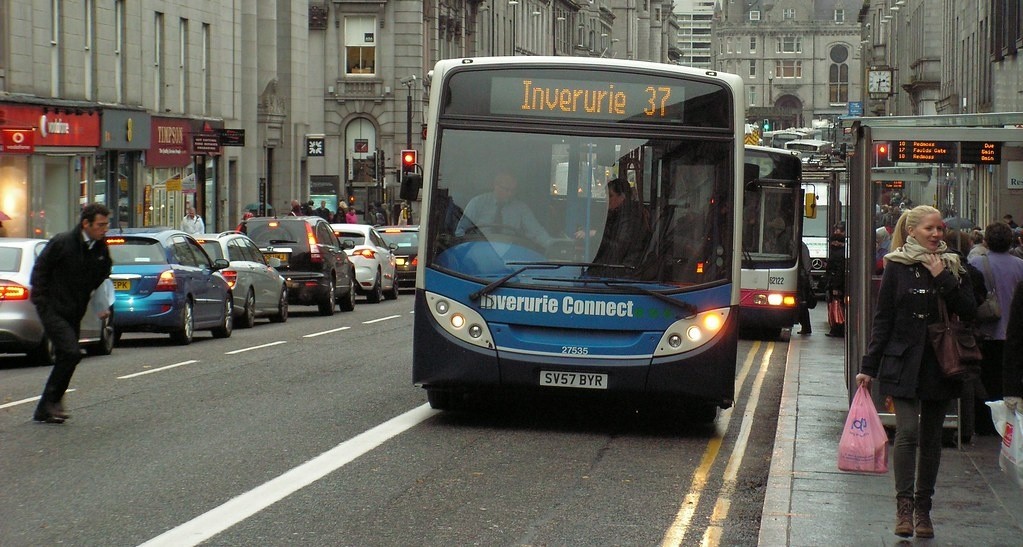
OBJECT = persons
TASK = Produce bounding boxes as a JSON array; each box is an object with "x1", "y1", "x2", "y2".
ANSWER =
[
  {"x1": 366, "y1": 201, "x2": 407, "y2": 225},
  {"x1": 288, "y1": 197, "x2": 367, "y2": 224},
  {"x1": 30, "y1": 203, "x2": 113, "y2": 424},
  {"x1": 799, "y1": 193, "x2": 1023, "y2": 442},
  {"x1": 180, "y1": 207, "x2": 204, "y2": 234},
  {"x1": 855, "y1": 204, "x2": 977, "y2": 537},
  {"x1": 454, "y1": 170, "x2": 553, "y2": 250},
  {"x1": 235, "y1": 210, "x2": 260, "y2": 235},
  {"x1": 574, "y1": 177, "x2": 649, "y2": 277}
]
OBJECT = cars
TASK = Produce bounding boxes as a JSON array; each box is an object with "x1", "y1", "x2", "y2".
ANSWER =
[
  {"x1": 329, "y1": 222, "x2": 400, "y2": 303},
  {"x1": 0, "y1": 238, "x2": 116, "y2": 364}
]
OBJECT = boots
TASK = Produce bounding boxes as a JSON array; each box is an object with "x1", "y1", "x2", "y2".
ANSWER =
[
  {"x1": 894, "y1": 497, "x2": 915, "y2": 536},
  {"x1": 915, "y1": 506, "x2": 934, "y2": 537}
]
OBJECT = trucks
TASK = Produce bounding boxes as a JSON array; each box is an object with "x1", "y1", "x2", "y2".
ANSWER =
[{"x1": 812, "y1": 119, "x2": 828, "y2": 129}]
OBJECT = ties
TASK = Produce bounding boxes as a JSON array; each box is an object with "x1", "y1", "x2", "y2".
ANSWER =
[{"x1": 492, "y1": 203, "x2": 503, "y2": 236}]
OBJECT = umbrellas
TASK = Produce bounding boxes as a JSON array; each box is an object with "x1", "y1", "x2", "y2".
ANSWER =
[{"x1": 942, "y1": 217, "x2": 974, "y2": 228}]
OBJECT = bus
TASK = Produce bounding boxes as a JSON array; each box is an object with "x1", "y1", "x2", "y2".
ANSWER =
[
  {"x1": 738, "y1": 144, "x2": 817, "y2": 344},
  {"x1": 771, "y1": 128, "x2": 823, "y2": 150},
  {"x1": 786, "y1": 140, "x2": 832, "y2": 158},
  {"x1": 400, "y1": 56, "x2": 760, "y2": 431}
]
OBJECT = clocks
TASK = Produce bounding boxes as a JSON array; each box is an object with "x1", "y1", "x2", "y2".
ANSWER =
[{"x1": 866, "y1": 67, "x2": 899, "y2": 100}]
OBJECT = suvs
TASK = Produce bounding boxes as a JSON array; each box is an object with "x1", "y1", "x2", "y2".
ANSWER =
[
  {"x1": 372, "y1": 225, "x2": 422, "y2": 294},
  {"x1": 237, "y1": 214, "x2": 356, "y2": 316},
  {"x1": 189, "y1": 230, "x2": 288, "y2": 326},
  {"x1": 103, "y1": 228, "x2": 234, "y2": 342}
]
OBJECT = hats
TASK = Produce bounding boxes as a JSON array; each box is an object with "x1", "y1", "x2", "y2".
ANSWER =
[{"x1": 828, "y1": 233, "x2": 844, "y2": 242}]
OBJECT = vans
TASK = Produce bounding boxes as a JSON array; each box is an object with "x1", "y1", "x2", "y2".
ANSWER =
[
  {"x1": 795, "y1": 183, "x2": 850, "y2": 296},
  {"x1": 552, "y1": 163, "x2": 595, "y2": 198}
]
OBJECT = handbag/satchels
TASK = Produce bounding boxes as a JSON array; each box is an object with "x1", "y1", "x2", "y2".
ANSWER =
[
  {"x1": 985, "y1": 398, "x2": 1023, "y2": 489},
  {"x1": 805, "y1": 283, "x2": 817, "y2": 309},
  {"x1": 838, "y1": 381, "x2": 888, "y2": 474},
  {"x1": 828, "y1": 300, "x2": 844, "y2": 327},
  {"x1": 929, "y1": 291, "x2": 982, "y2": 375},
  {"x1": 976, "y1": 252, "x2": 1002, "y2": 321}
]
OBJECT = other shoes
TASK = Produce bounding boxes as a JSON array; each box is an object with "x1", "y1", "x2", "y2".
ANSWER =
[
  {"x1": 33, "y1": 403, "x2": 69, "y2": 423},
  {"x1": 797, "y1": 328, "x2": 811, "y2": 334},
  {"x1": 825, "y1": 332, "x2": 843, "y2": 338}
]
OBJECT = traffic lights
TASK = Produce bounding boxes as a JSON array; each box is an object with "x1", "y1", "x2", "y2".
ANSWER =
[
  {"x1": 366, "y1": 151, "x2": 377, "y2": 181},
  {"x1": 400, "y1": 149, "x2": 417, "y2": 182},
  {"x1": 876, "y1": 143, "x2": 890, "y2": 167},
  {"x1": 764, "y1": 119, "x2": 769, "y2": 131}
]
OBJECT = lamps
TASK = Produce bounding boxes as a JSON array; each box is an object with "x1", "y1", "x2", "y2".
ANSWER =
[
  {"x1": 881, "y1": 0, "x2": 905, "y2": 23},
  {"x1": 508, "y1": 1, "x2": 619, "y2": 43}
]
